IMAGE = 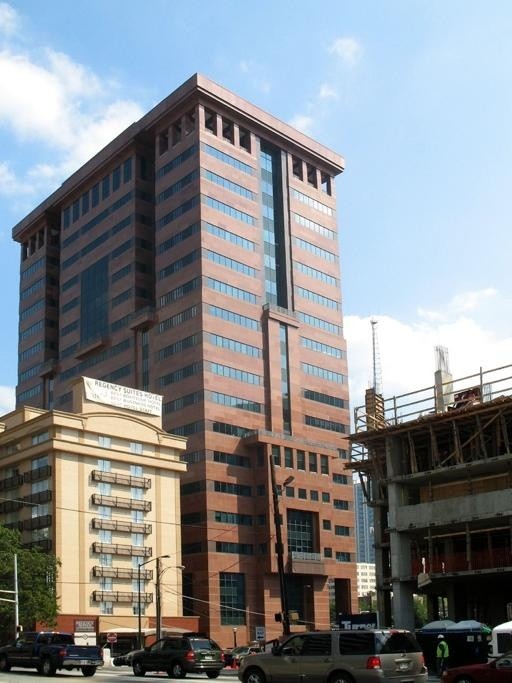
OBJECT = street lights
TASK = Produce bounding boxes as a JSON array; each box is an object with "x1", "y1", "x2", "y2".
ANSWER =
[
  {"x1": 138, "y1": 555, "x2": 170, "y2": 650},
  {"x1": 156, "y1": 557, "x2": 186, "y2": 642}
]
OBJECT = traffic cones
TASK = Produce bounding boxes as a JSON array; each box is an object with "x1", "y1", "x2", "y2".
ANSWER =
[{"x1": 231, "y1": 658, "x2": 238, "y2": 669}]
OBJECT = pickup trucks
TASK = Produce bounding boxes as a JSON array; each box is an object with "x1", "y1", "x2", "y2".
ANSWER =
[{"x1": 0, "y1": 631, "x2": 104, "y2": 677}]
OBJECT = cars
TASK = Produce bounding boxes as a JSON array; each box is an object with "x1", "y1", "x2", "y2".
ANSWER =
[
  {"x1": 442, "y1": 650, "x2": 512, "y2": 683},
  {"x1": 114, "y1": 648, "x2": 145, "y2": 666}
]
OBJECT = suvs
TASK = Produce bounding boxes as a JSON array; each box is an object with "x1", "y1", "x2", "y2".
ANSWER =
[
  {"x1": 225, "y1": 646, "x2": 262, "y2": 666},
  {"x1": 131, "y1": 636, "x2": 224, "y2": 678},
  {"x1": 238, "y1": 630, "x2": 429, "y2": 683}
]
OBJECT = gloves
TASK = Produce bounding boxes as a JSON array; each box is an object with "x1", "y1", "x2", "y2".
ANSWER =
[{"x1": 440, "y1": 658, "x2": 444, "y2": 663}]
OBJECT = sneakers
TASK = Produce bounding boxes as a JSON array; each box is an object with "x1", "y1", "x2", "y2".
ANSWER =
[{"x1": 436, "y1": 676, "x2": 441, "y2": 678}]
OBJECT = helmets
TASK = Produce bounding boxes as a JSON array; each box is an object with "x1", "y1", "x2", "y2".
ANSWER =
[{"x1": 438, "y1": 634, "x2": 444, "y2": 638}]
